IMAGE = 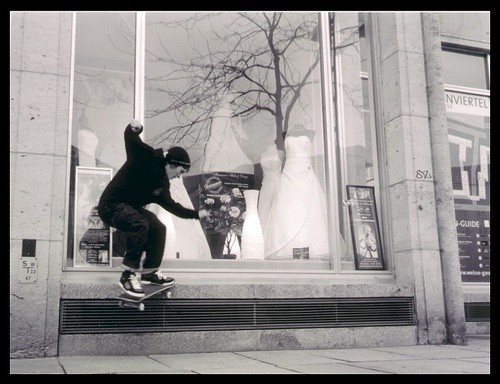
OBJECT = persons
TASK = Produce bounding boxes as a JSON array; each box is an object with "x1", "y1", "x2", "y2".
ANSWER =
[{"x1": 97, "y1": 120, "x2": 212, "y2": 298}]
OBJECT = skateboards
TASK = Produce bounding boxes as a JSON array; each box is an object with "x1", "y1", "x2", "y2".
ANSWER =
[{"x1": 115, "y1": 277, "x2": 177, "y2": 311}]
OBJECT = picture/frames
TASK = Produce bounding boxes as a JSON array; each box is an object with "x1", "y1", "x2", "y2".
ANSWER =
[
  {"x1": 346, "y1": 183, "x2": 384, "y2": 269},
  {"x1": 73, "y1": 166, "x2": 114, "y2": 269}
]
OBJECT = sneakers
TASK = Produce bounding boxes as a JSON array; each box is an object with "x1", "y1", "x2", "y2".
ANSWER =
[
  {"x1": 118, "y1": 270, "x2": 145, "y2": 298},
  {"x1": 140, "y1": 270, "x2": 175, "y2": 286}
]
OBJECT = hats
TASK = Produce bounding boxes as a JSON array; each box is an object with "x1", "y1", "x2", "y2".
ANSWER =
[{"x1": 166, "y1": 147, "x2": 190, "y2": 167}]
